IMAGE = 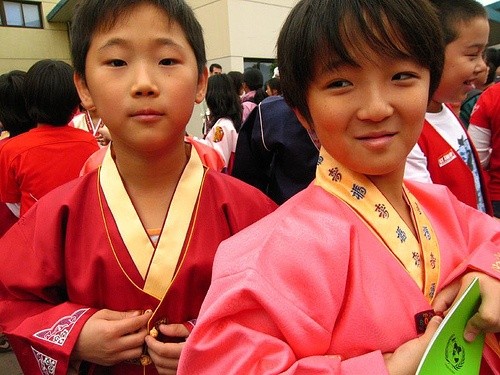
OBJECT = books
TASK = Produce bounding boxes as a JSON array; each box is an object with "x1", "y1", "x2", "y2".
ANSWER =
[{"x1": 414, "y1": 277, "x2": 486, "y2": 375}]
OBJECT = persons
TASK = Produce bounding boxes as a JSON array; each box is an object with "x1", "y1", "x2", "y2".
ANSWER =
[
  {"x1": 177, "y1": 0, "x2": 500, "y2": 375},
  {"x1": 403, "y1": 0, "x2": 500, "y2": 216},
  {"x1": 0, "y1": 57, "x2": 116, "y2": 352},
  {"x1": 0, "y1": 0, "x2": 280, "y2": 375},
  {"x1": 185, "y1": 62, "x2": 321, "y2": 209}
]
